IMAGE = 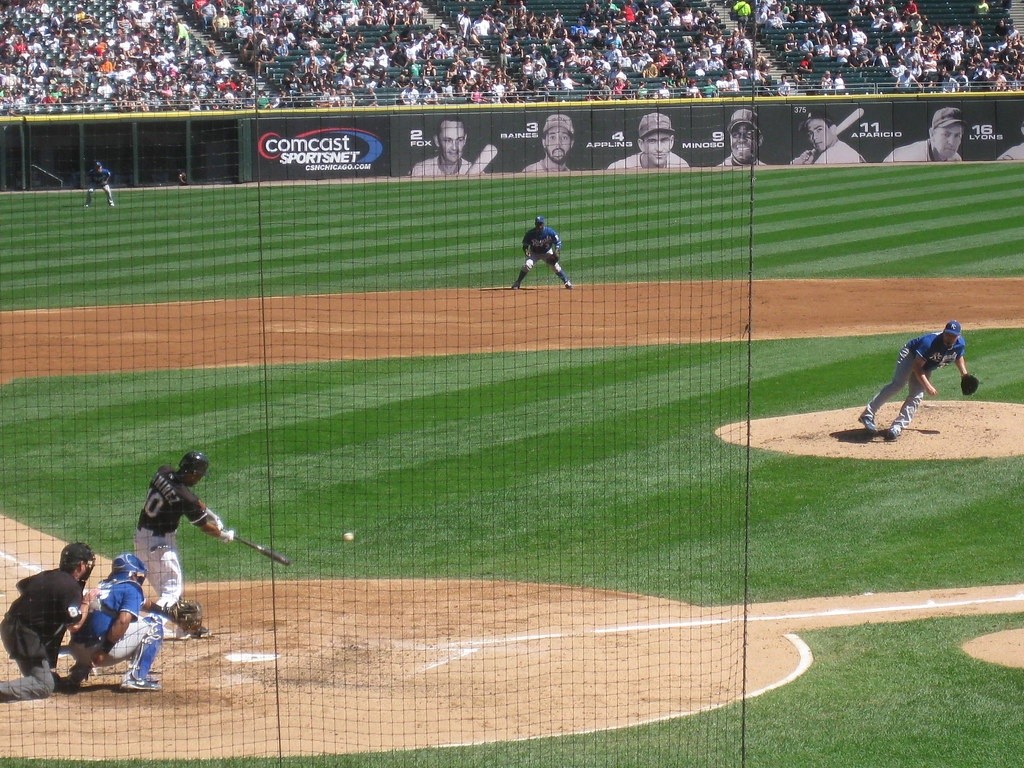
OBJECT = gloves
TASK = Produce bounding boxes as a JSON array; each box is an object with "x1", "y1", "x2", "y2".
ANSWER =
[
  {"x1": 210, "y1": 516, "x2": 222, "y2": 531},
  {"x1": 220, "y1": 530, "x2": 234, "y2": 543}
]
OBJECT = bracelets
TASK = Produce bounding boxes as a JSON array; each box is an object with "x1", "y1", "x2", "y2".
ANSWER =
[{"x1": 82, "y1": 602, "x2": 89, "y2": 605}]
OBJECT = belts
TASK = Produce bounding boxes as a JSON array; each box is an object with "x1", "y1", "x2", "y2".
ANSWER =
[
  {"x1": 136, "y1": 524, "x2": 165, "y2": 536},
  {"x1": 70, "y1": 635, "x2": 102, "y2": 642}
]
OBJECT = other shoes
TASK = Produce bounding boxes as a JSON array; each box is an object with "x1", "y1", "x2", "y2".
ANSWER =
[
  {"x1": 512, "y1": 285, "x2": 519, "y2": 289},
  {"x1": 164, "y1": 627, "x2": 177, "y2": 639},
  {"x1": 148, "y1": 602, "x2": 169, "y2": 617},
  {"x1": 565, "y1": 283, "x2": 573, "y2": 289},
  {"x1": 51, "y1": 672, "x2": 60, "y2": 679}
]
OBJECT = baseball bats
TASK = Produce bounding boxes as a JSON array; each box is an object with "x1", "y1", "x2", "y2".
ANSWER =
[
  {"x1": 466, "y1": 144, "x2": 498, "y2": 174},
  {"x1": 808, "y1": 108, "x2": 865, "y2": 157},
  {"x1": 222, "y1": 528, "x2": 291, "y2": 566}
]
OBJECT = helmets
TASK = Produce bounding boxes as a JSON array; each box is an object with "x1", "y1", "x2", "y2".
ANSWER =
[{"x1": 179, "y1": 452, "x2": 211, "y2": 476}]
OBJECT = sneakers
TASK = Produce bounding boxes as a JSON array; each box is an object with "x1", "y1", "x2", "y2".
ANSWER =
[
  {"x1": 884, "y1": 428, "x2": 896, "y2": 439},
  {"x1": 56, "y1": 678, "x2": 81, "y2": 692},
  {"x1": 858, "y1": 414, "x2": 876, "y2": 433},
  {"x1": 121, "y1": 677, "x2": 162, "y2": 690}
]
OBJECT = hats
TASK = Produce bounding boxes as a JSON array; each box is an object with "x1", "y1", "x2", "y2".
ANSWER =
[
  {"x1": 61, "y1": 542, "x2": 95, "y2": 565},
  {"x1": 728, "y1": 109, "x2": 762, "y2": 134},
  {"x1": 798, "y1": 111, "x2": 833, "y2": 132},
  {"x1": 638, "y1": 113, "x2": 674, "y2": 137},
  {"x1": 931, "y1": 108, "x2": 969, "y2": 128},
  {"x1": 535, "y1": 216, "x2": 543, "y2": 224},
  {"x1": 945, "y1": 320, "x2": 961, "y2": 336},
  {"x1": 543, "y1": 115, "x2": 575, "y2": 134},
  {"x1": 108, "y1": 553, "x2": 147, "y2": 583}
]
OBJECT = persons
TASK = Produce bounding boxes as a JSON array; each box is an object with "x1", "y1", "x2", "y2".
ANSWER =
[
  {"x1": 716, "y1": 109, "x2": 766, "y2": 167},
  {"x1": 84, "y1": 161, "x2": 115, "y2": 207},
  {"x1": 511, "y1": 216, "x2": 573, "y2": 290},
  {"x1": 607, "y1": 113, "x2": 689, "y2": 170},
  {"x1": 523, "y1": 114, "x2": 577, "y2": 173},
  {"x1": 0, "y1": 542, "x2": 101, "y2": 703},
  {"x1": 134, "y1": 452, "x2": 233, "y2": 641},
  {"x1": 406, "y1": 117, "x2": 485, "y2": 177},
  {"x1": 859, "y1": 320, "x2": 978, "y2": 439},
  {"x1": 789, "y1": 111, "x2": 865, "y2": 165},
  {"x1": 997, "y1": 122, "x2": 1024, "y2": 160},
  {"x1": 884, "y1": 107, "x2": 967, "y2": 163},
  {"x1": 66, "y1": 554, "x2": 175, "y2": 692},
  {"x1": 0, "y1": 0, "x2": 1024, "y2": 117}
]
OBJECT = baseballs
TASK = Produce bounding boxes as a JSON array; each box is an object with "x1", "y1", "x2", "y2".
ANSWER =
[{"x1": 343, "y1": 532, "x2": 354, "y2": 543}]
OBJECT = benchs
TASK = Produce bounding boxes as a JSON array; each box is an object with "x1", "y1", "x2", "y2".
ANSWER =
[{"x1": 0, "y1": 0, "x2": 1024, "y2": 116}]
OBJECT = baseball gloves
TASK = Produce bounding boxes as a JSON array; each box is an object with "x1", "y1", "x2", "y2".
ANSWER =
[
  {"x1": 169, "y1": 599, "x2": 202, "y2": 635},
  {"x1": 546, "y1": 253, "x2": 560, "y2": 266},
  {"x1": 961, "y1": 374, "x2": 979, "y2": 395}
]
